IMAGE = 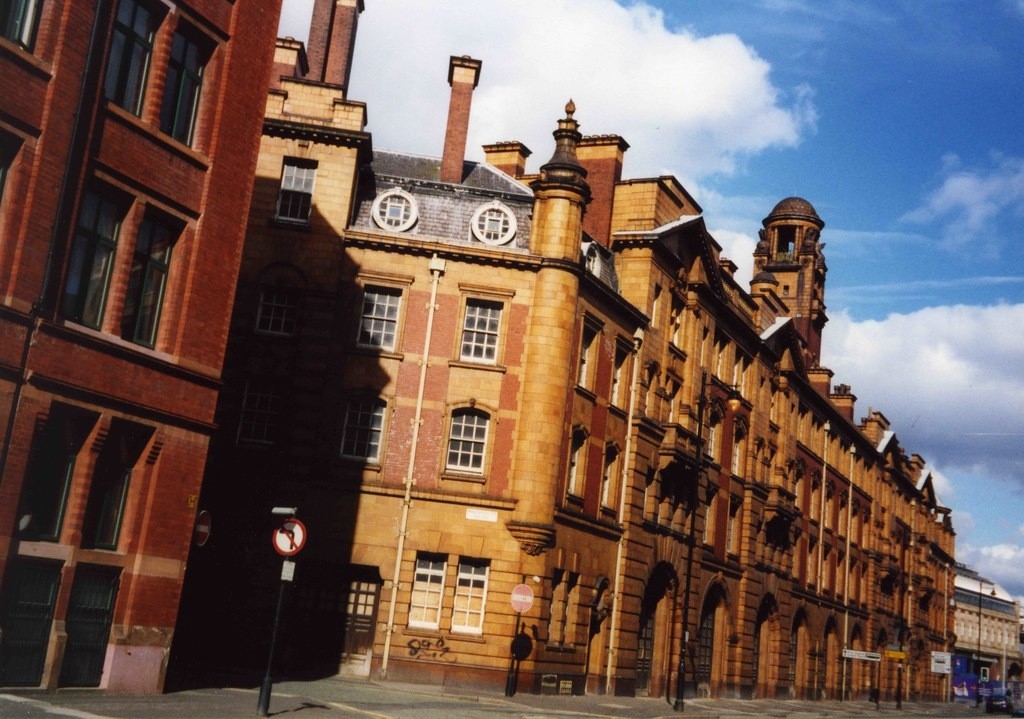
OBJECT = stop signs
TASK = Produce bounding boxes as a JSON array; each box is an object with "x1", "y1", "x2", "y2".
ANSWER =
[
  {"x1": 194, "y1": 509, "x2": 215, "y2": 549},
  {"x1": 510, "y1": 582, "x2": 536, "y2": 614}
]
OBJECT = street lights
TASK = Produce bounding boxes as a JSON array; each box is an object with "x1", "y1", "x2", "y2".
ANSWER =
[
  {"x1": 671, "y1": 371, "x2": 743, "y2": 712},
  {"x1": 896, "y1": 525, "x2": 929, "y2": 706},
  {"x1": 975, "y1": 581, "x2": 996, "y2": 709}
]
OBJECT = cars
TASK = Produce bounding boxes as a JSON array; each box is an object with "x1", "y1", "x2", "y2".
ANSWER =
[{"x1": 986, "y1": 694, "x2": 1014, "y2": 716}]
